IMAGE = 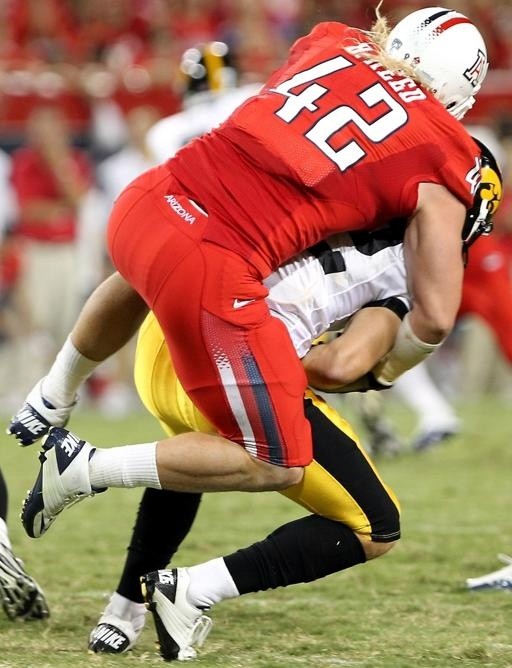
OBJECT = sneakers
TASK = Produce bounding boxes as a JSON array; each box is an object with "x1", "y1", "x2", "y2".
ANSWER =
[
  {"x1": 139, "y1": 567, "x2": 213, "y2": 662},
  {"x1": 87, "y1": 615, "x2": 143, "y2": 656},
  {"x1": 19, "y1": 426, "x2": 107, "y2": 538},
  {"x1": 463, "y1": 553, "x2": 512, "y2": 592},
  {"x1": 0, "y1": 541, "x2": 50, "y2": 625},
  {"x1": 6, "y1": 376, "x2": 78, "y2": 447}
]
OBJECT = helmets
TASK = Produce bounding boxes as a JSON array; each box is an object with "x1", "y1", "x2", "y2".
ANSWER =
[
  {"x1": 461, "y1": 138, "x2": 504, "y2": 267},
  {"x1": 382, "y1": 7, "x2": 487, "y2": 121},
  {"x1": 181, "y1": 41, "x2": 239, "y2": 97}
]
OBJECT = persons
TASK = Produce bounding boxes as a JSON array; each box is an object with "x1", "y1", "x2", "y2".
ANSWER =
[
  {"x1": 0, "y1": 469, "x2": 50, "y2": 627},
  {"x1": 4, "y1": 6, "x2": 493, "y2": 540},
  {"x1": 3, "y1": 5, "x2": 511, "y2": 379},
  {"x1": 87, "y1": 133, "x2": 506, "y2": 664},
  {"x1": 148, "y1": 40, "x2": 462, "y2": 456}
]
